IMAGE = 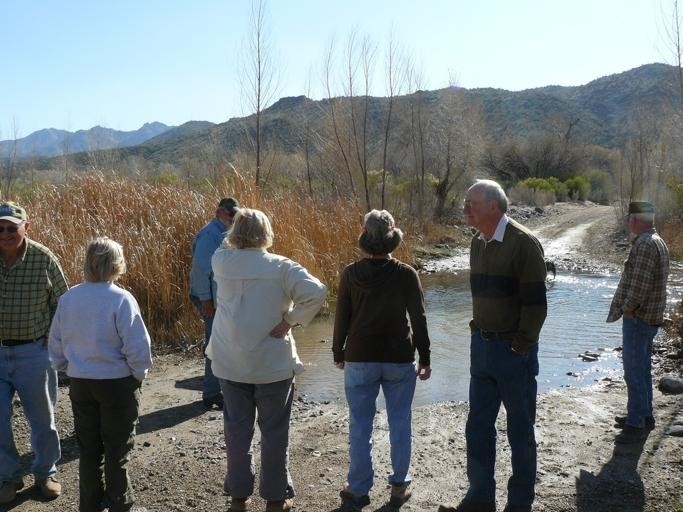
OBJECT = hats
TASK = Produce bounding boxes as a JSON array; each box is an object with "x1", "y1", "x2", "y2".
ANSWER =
[
  {"x1": 624, "y1": 202, "x2": 654, "y2": 217},
  {"x1": 218, "y1": 197, "x2": 240, "y2": 214},
  {"x1": 0, "y1": 202, "x2": 28, "y2": 225}
]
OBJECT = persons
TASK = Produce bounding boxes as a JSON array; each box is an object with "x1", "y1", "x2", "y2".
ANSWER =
[
  {"x1": 204, "y1": 208, "x2": 329, "y2": 511},
  {"x1": 604, "y1": 199, "x2": 670, "y2": 444},
  {"x1": 186, "y1": 198, "x2": 237, "y2": 407},
  {"x1": 0, "y1": 202, "x2": 69, "y2": 505},
  {"x1": 46, "y1": 238, "x2": 152, "y2": 512},
  {"x1": 330, "y1": 207, "x2": 433, "y2": 501},
  {"x1": 438, "y1": 178, "x2": 548, "y2": 511}
]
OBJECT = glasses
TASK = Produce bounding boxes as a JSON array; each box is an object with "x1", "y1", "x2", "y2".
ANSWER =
[{"x1": 0, "y1": 222, "x2": 23, "y2": 232}]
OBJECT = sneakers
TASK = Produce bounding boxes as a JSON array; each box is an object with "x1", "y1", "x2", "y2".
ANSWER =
[
  {"x1": 390, "y1": 485, "x2": 412, "y2": 506},
  {"x1": 266, "y1": 499, "x2": 293, "y2": 511},
  {"x1": 615, "y1": 416, "x2": 655, "y2": 444},
  {"x1": 231, "y1": 497, "x2": 252, "y2": 510},
  {"x1": 344, "y1": 482, "x2": 370, "y2": 505}
]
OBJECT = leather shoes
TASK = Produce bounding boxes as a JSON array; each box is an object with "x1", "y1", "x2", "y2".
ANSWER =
[
  {"x1": 0, "y1": 482, "x2": 21, "y2": 504},
  {"x1": 438, "y1": 501, "x2": 496, "y2": 512},
  {"x1": 40, "y1": 477, "x2": 61, "y2": 497}
]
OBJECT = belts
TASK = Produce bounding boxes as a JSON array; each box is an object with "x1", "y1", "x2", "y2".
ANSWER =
[
  {"x1": 0, "y1": 336, "x2": 44, "y2": 348},
  {"x1": 479, "y1": 329, "x2": 516, "y2": 342}
]
